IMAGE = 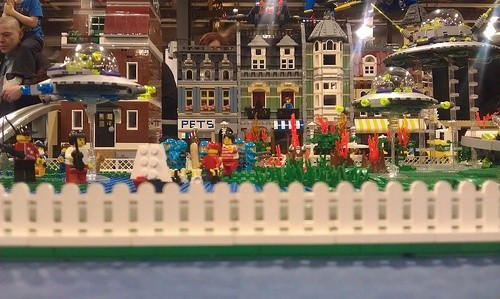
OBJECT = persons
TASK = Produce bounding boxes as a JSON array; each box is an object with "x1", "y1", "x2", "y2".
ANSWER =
[
  {"x1": 65, "y1": 133, "x2": 94, "y2": 183},
  {"x1": 0, "y1": 1, "x2": 46, "y2": 88},
  {"x1": 13, "y1": 124, "x2": 39, "y2": 182},
  {"x1": 34, "y1": 139, "x2": 47, "y2": 176},
  {"x1": 0, "y1": 16, "x2": 45, "y2": 117},
  {"x1": 163, "y1": 128, "x2": 257, "y2": 181},
  {"x1": 283, "y1": 97, "x2": 293, "y2": 109},
  {"x1": 407, "y1": 142, "x2": 414, "y2": 157},
  {"x1": 58, "y1": 147, "x2": 67, "y2": 172}
]
